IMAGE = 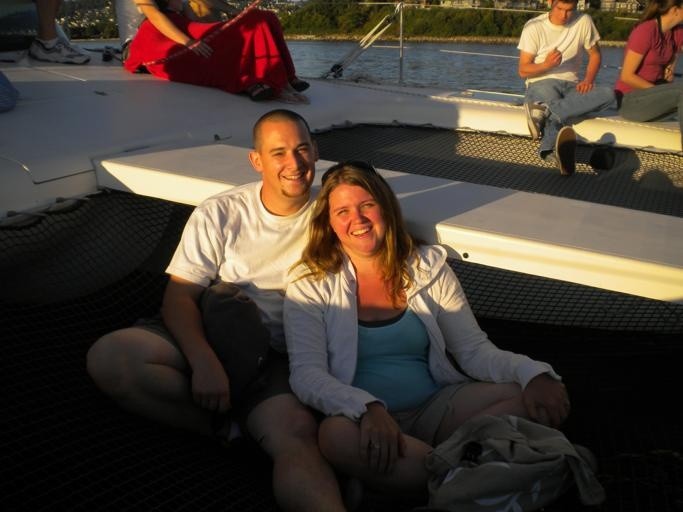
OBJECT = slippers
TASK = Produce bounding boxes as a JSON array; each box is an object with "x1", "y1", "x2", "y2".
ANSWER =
[
  {"x1": 292, "y1": 78, "x2": 310, "y2": 92},
  {"x1": 247, "y1": 82, "x2": 274, "y2": 102}
]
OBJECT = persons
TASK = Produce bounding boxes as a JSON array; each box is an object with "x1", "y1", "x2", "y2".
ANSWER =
[
  {"x1": 518, "y1": 0, "x2": 617, "y2": 176},
  {"x1": 614, "y1": 0, "x2": 683, "y2": 133}
]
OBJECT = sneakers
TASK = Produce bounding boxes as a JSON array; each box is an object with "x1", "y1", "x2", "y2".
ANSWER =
[
  {"x1": 524, "y1": 101, "x2": 551, "y2": 141},
  {"x1": 556, "y1": 125, "x2": 576, "y2": 176}
]
